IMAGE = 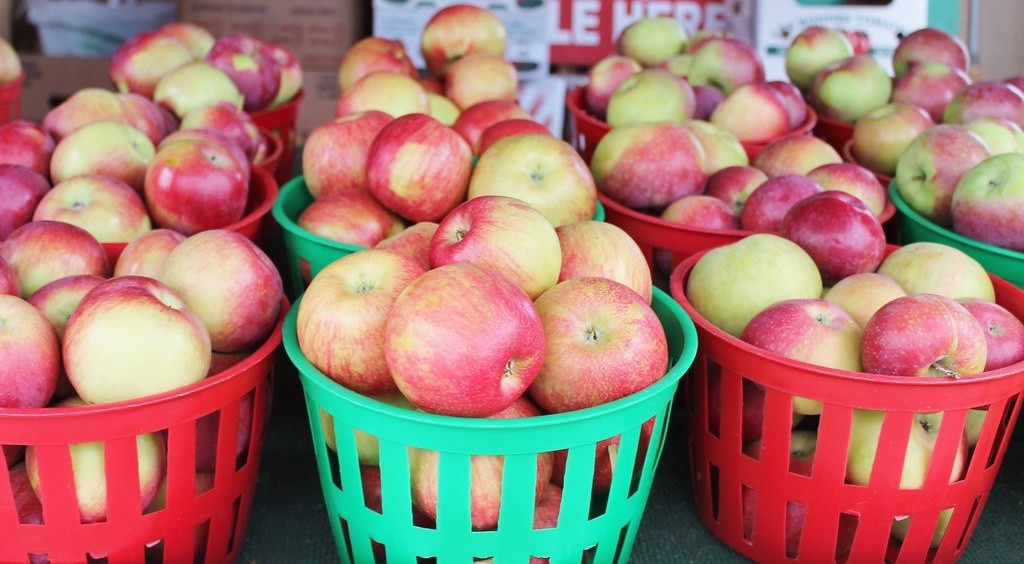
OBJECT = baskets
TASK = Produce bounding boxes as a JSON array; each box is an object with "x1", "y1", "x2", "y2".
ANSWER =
[{"x1": 0, "y1": 86, "x2": 1024, "y2": 564}]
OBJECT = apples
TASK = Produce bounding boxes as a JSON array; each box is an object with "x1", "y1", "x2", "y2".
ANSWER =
[{"x1": 0, "y1": 4, "x2": 1024, "y2": 564}]
